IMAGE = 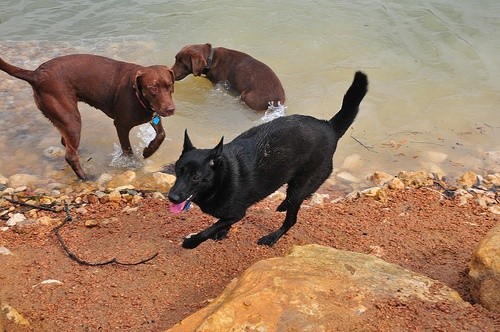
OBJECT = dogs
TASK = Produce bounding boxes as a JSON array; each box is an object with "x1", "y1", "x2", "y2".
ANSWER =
[
  {"x1": 169, "y1": 43, "x2": 285, "y2": 111},
  {"x1": 0, "y1": 54, "x2": 175, "y2": 181},
  {"x1": 168, "y1": 70, "x2": 370, "y2": 249}
]
